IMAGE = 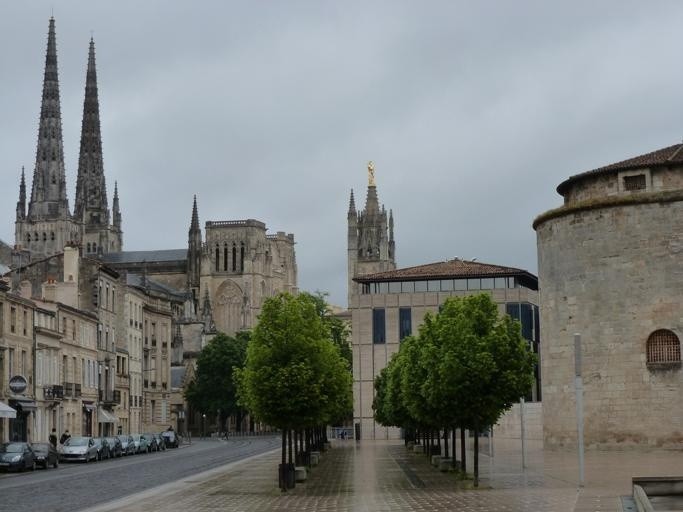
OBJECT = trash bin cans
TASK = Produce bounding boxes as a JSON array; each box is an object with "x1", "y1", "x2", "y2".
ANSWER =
[
  {"x1": 279, "y1": 464, "x2": 294, "y2": 488},
  {"x1": 296, "y1": 451, "x2": 310, "y2": 473}
]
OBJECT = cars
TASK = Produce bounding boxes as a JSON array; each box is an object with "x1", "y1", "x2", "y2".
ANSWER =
[
  {"x1": 0, "y1": 441, "x2": 38, "y2": 472},
  {"x1": 93, "y1": 431, "x2": 179, "y2": 460},
  {"x1": 31, "y1": 442, "x2": 58, "y2": 469},
  {"x1": 57, "y1": 436, "x2": 99, "y2": 463}
]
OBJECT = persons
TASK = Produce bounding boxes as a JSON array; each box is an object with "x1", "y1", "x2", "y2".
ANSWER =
[
  {"x1": 367, "y1": 159, "x2": 375, "y2": 185},
  {"x1": 167, "y1": 424, "x2": 174, "y2": 431},
  {"x1": 341, "y1": 429, "x2": 348, "y2": 440},
  {"x1": 60, "y1": 430, "x2": 71, "y2": 444},
  {"x1": 48, "y1": 428, "x2": 57, "y2": 448}
]
